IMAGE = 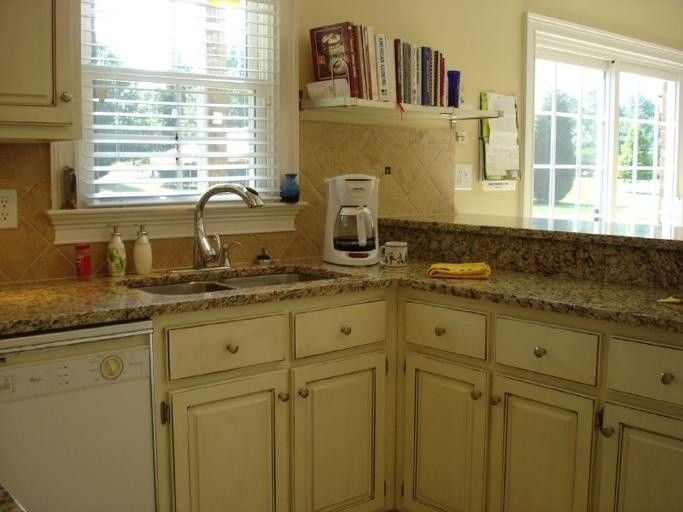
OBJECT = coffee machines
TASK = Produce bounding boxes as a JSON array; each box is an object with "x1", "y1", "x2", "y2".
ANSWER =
[{"x1": 322, "y1": 174, "x2": 381, "y2": 268}]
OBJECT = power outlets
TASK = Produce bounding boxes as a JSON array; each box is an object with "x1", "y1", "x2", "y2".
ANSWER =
[{"x1": 0, "y1": 189, "x2": 17, "y2": 230}]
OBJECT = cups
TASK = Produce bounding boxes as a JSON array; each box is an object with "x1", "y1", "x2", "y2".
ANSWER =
[{"x1": 379, "y1": 241, "x2": 409, "y2": 268}]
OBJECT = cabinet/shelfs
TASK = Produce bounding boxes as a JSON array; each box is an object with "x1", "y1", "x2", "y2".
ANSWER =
[
  {"x1": 155, "y1": 286, "x2": 395, "y2": 510},
  {"x1": 603, "y1": 321, "x2": 683, "y2": 511},
  {"x1": 0, "y1": 1, "x2": 81, "y2": 144},
  {"x1": 392, "y1": 284, "x2": 604, "y2": 512}
]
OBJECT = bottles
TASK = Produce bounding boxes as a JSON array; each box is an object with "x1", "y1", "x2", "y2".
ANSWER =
[
  {"x1": 74, "y1": 242, "x2": 92, "y2": 282},
  {"x1": 104, "y1": 224, "x2": 127, "y2": 277},
  {"x1": 279, "y1": 173, "x2": 300, "y2": 204},
  {"x1": 63, "y1": 167, "x2": 77, "y2": 209}
]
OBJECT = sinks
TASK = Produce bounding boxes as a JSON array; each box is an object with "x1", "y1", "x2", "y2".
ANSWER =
[
  {"x1": 112, "y1": 270, "x2": 237, "y2": 298},
  {"x1": 214, "y1": 258, "x2": 353, "y2": 291}
]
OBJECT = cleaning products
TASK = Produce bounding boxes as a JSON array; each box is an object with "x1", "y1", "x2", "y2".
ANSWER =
[{"x1": 107, "y1": 225, "x2": 127, "y2": 277}]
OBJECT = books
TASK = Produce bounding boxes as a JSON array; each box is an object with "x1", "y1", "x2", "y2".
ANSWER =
[{"x1": 309, "y1": 22, "x2": 460, "y2": 108}]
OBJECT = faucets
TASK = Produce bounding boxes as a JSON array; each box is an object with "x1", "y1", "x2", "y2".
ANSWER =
[{"x1": 192, "y1": 183, "x2": 264, "y2": 270}]
